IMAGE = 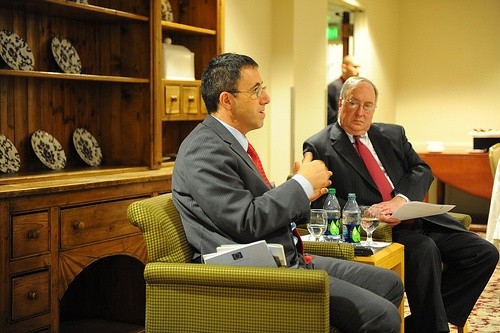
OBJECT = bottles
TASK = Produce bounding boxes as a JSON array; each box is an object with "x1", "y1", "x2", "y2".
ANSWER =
[
  {"x1": 341, "y1": 193, "x2": 361, "y2": 247},
  {"x1": 322, "y1": 188, "x2": 341, "y2": 243}
]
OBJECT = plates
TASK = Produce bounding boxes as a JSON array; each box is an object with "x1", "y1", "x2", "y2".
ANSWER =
[
  {"x1": 161, "y1": 0, "x2": 174, "y2": 22},
  {"x1": 72, "y1": 128, "x2": 102, "y2": 166},
  {"x1": 51, "y1": 35, "x2": 82, "y2": 73},
  {"x1": 31, "y1": 130, "x2": 67, "y2": 170},
  {"x1": 0, "y1": 30, "x2": 35, "y2": 71},
  {"x1": 0, "y1": 133, "x2": 21, "y2": 173}
]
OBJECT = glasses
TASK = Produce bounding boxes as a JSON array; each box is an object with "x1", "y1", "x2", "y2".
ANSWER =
[
  {"x1": 344, "y1": 99, "x2": 375, "y2": 113},
  {"x1": 226, "y1": 84, "x2": 267, "y2": 98}
]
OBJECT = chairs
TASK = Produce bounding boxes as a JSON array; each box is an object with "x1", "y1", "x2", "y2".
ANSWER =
[
  {"x1": 295, "y1": 212, "x2": 472, "y2": 332},
  {"x1": 127, "y1": 193, "x2": 359, "y2": 332}
]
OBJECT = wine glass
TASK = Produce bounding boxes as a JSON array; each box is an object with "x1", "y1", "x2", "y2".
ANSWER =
[
  {"x1": 307, "y1": 208, "x2": 328, "y2": 241},
  {"x1": 360, "y1": 206, "x2": 381, "y2": 247}
]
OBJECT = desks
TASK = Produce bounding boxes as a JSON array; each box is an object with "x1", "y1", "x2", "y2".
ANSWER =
[{"x1": 415, "y1": 146, "x2": 494, "y2": 232}]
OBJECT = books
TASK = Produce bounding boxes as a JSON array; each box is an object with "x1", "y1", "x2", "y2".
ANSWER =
[
  {"x1": 216, "y1": 244, "x2": 287, "y2": 266},
  {"x1": 205, "y1": 239, "x2": 278, "y2": 268}
]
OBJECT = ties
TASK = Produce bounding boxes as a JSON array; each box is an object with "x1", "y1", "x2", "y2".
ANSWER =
[
  {"x1": 353, "y1": 134, "x2": 414, "y2": 225},
  {"x1": 247, "y1": 143, "x2": 313, "y2": 264}
]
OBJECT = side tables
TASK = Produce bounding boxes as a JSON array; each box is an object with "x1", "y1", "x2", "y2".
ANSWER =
[{"x1": 299, "y1": 234, "x2": 405, "y2": 332}]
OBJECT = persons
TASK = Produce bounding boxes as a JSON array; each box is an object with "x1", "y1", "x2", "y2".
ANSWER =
[
  {"x1": 303, "y1": 76, "x2": 499, "y2": 333},
  {"x1": 327, "y1": 55, "x2": 361, "y2": 126},
  {"x1": 172, "y1": 52, "x2": 404, "y2": 333}
]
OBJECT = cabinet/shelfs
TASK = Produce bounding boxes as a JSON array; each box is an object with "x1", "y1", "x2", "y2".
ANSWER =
[
  {"x1": 0, "y1": 169, "x2": 174, "y2": 333},
  {"x1": 1, "y1": 0, "x2": 226, "y2": 186}
]
